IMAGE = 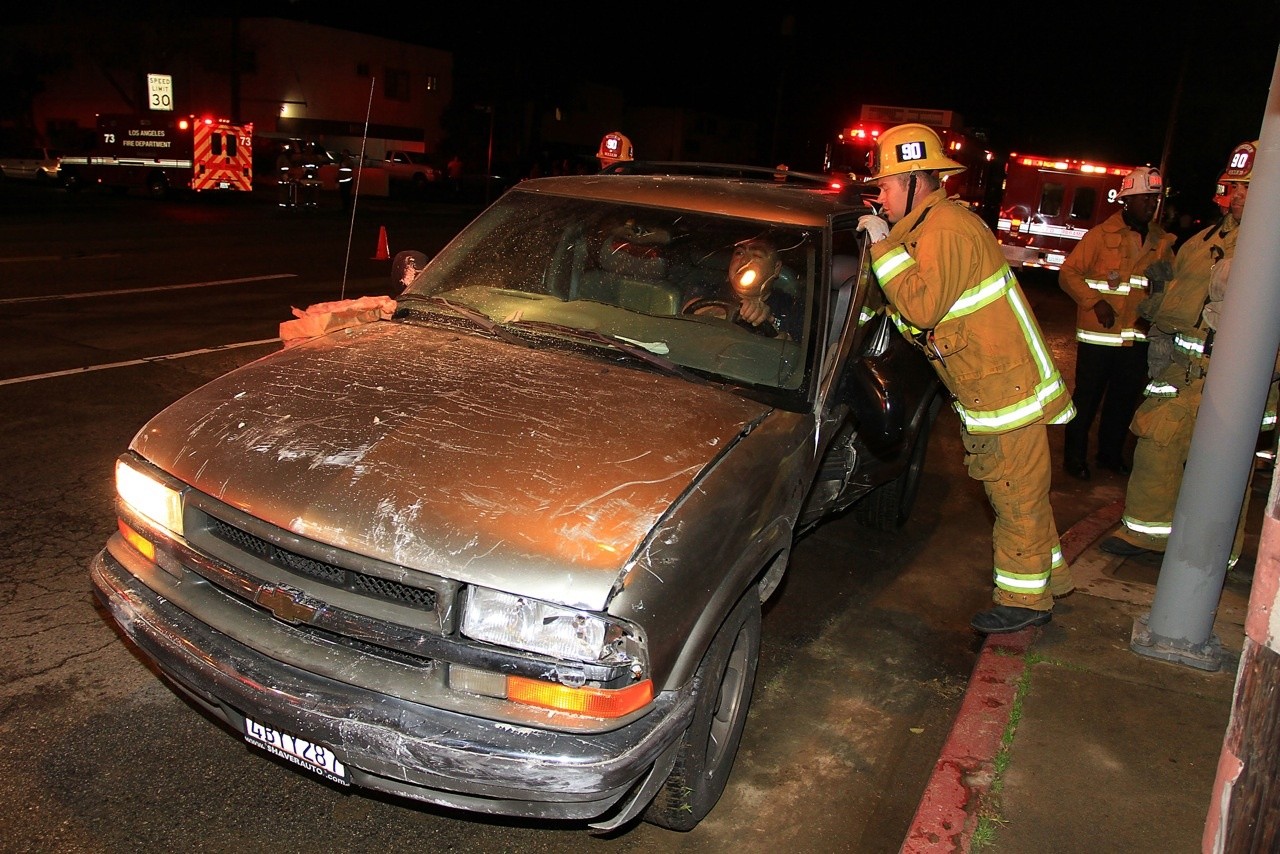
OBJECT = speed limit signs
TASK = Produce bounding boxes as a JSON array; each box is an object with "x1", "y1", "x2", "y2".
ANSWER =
[{"x1": 148, "y1": 73, "x2": 172, "y2": 111}]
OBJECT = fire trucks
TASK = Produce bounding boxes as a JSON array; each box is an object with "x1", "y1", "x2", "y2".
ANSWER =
[
  {"x1": 824, "y1": 105, "x2": 1162, "y2": 274},
  {"x1": 37, "y1": 113, "x2": 255, "y2": 203}
]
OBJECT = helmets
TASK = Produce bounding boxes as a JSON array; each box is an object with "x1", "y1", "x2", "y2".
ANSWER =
[
  {"x1": 596, "y1": 132, "x2": 633, "y2": 162},
  {"x1": 1116, "y1": 167, "x2": 1164, "y2": 201},
  {"x1": 862, "y1": 123, "x2": 968, "y2": 182},
  {"x1": 1220, "y1": 139, "x2": 1260, "y2": 184},
  {"x1": 1213, "y1": 173, "x2": 1233, "y2": 207}
]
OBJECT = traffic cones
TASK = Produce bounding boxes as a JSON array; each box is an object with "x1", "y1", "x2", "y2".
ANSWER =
[{"x1": 369, "y1": 225, "x2": 391, "y2": 261}]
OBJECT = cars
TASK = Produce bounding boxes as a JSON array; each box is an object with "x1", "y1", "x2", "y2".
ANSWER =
[
  {"x1": 88, "y1": 158, "x2": 948, "y2": 836},
  {"x1": 0, "y1": 145, "x2": 71, "y2": 188},
  {"x1": 255, "y1": 132, "x2": 333, "y2": 188}
]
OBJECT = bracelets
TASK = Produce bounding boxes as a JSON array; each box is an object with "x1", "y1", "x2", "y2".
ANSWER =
[{"x1": 871, "y1": 233, "x2": 887, "y2": 246}]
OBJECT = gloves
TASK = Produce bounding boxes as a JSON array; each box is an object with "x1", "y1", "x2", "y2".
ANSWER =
[{"x1": 855, "y1": 215, "x2": 890, "y2": 246}]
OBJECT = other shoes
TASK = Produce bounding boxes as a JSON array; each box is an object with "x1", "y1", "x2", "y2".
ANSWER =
[
  {"x1": 1067, "y1": 458, "x2": 1091, "y2": 479},
  {"x1": 1098, "y1": 457, "x2": 1131, "y2": 477},
  {"x1": 972, "y1": 602, "x2": 1052, "y2": 634},
  {"x1": 1100, "y1": 535, "x2": 1145, "y2": 556}
]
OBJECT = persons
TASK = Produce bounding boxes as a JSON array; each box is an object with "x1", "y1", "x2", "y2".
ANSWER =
[
  {"x1": 301, "y1": 143, "x2": 324, "y2": 208},
  {"x1": 684, "y1": 228, "x2": 793, "y2": 340},
  {"x1": 610, "y1": 207, "x2": 678, "y2": 253},
  {"x1": 1214, "y1": 173, "x2": 1233, "y2": 216},
  {"x1": 1059, "y1": 167, "x2": 1175, "y2": 480},
  {"x1": 448, "y1": 155, "x2": 463, "y2": 196},
  {"x1": 581, "y1": 132, "x2": 661, "y2": 257},
  {"x1": 335, "y1": 149, "x2": 354, "y2": 194},
  {"x1": 856, "y1": 123, "x2": 1076, "y2": 634},
  {"x1": 1098, "y1": 137, "x2": 1280, "y2": 575},
  {"x1": 276, "y1": 143, "x2": 297, "y2": 206}
]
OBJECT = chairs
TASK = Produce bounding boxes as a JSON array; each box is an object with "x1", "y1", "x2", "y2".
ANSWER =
[{"x1": 578, "y1": 223, "x2": 680, "y2": 316}]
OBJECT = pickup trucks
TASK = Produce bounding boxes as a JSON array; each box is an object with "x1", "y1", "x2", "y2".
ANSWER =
[{"x1": 353, "y1": 148, "x2": 449, "y2": 193}]
